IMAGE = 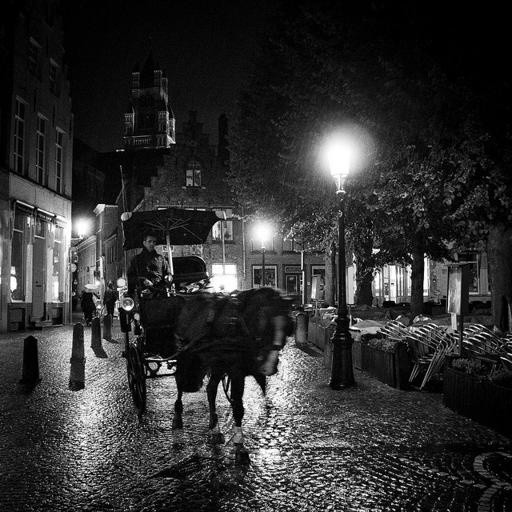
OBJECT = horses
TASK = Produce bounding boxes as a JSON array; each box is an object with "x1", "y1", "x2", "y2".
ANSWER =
[{"x1": 158, "y1": 286, "x2": 299, "y2": 445}]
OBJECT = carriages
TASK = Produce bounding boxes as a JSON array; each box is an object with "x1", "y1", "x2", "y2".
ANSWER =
[{"x1": 118, "y1": 255, "x2": 297, "y2": 443}]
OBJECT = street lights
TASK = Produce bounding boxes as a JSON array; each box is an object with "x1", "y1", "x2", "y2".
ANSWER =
[
  {"x1": 311, "y1": 123, "x2": 380, "y2": 389},
  {"x1": 245, "y1": 214, "x2": 279, "y2": 289}
]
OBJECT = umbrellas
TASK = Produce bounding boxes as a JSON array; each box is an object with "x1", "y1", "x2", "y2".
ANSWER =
[
  {"x1": 81, "y1": 282, "x2": 101, "y2": 293},
  {"x1": 120, "y1": 205, "x2": 224, "y2": 298}
]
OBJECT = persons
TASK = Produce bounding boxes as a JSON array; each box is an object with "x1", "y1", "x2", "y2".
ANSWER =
[
  {"x1": 129, "y1": 233, "x2": 173, "y2": 301},
  {"x1": 102, "y1": 281, "x2": 119, "y2": 321},
  {"x1": 79, "y1": 291, "x2": 101, "y2": 327}
]
